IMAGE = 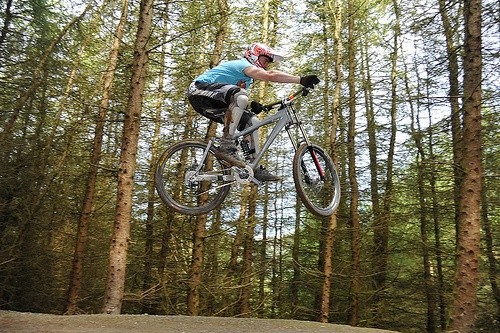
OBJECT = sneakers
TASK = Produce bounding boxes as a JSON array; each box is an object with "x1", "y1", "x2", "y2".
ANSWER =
[
  {"x1": 244, "y1": 165, "x2": 280, "y2": 182},
  {"x1": 216, "y1": 144, "x2": 247, "y2": 168}
]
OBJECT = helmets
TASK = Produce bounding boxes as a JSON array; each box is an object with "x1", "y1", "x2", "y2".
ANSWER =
[{"x1": 244, "y1": 43, "x2": 274, "y2": 70}]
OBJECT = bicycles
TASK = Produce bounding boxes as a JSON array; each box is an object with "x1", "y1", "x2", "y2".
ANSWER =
[{"x1": 155, "y1": 77, "x2": 341, "y2": 217}]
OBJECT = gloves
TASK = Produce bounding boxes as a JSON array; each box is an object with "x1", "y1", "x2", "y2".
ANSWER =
[
  {"x1": 300, "y1": 74, "x2": 319, "y2": 90},
  {"x1": 250, "y1": 101, "x2": 263, "y2": 114}
]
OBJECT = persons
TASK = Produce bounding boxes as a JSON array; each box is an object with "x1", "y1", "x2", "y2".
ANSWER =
[{"x1": 187, "y1": 43, "x2": 321, "y2": 181}]
